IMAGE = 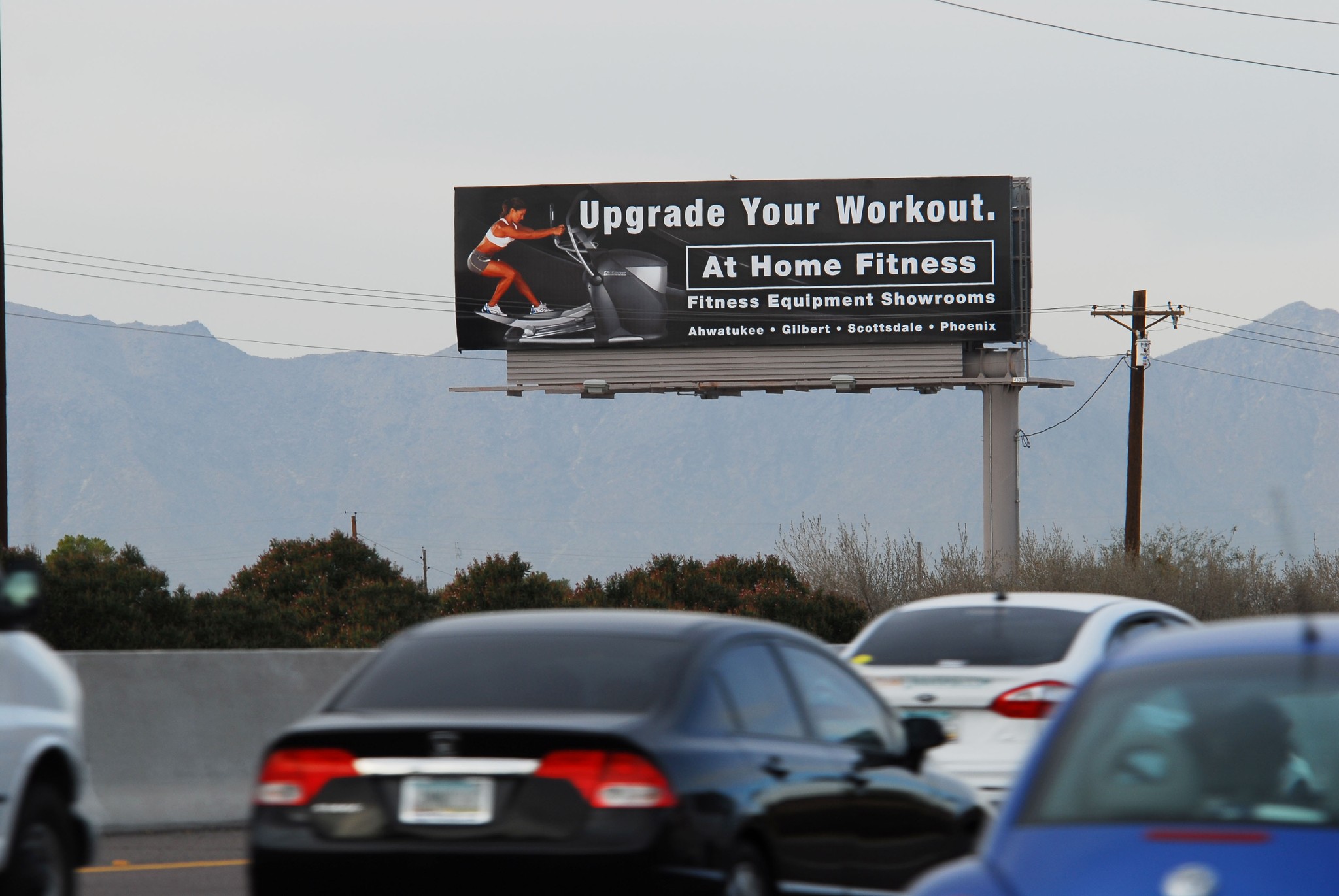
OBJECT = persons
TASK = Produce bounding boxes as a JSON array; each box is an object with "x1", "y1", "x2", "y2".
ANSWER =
[{"x1": 465, "y1": 198, "x2": 568, "y2": 319}]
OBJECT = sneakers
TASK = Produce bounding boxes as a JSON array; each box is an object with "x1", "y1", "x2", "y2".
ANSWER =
[
  {"x1": 530, "y1": 303, "x2": 554, "y2": 314},
  {"x1": 481, "y1": 303, "x2": 508, "y2": 317}
]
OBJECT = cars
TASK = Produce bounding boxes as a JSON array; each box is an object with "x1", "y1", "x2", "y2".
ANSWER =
[
  {"x1": 255, "y1": 594, "x2": 994, "y2": 894},
  {"x1": 904, "y1": 605, "x2": 1339, "y2": 896},
  {"x1": 839, "y1": 591, "x2": 1207, "y2": 823}
]
